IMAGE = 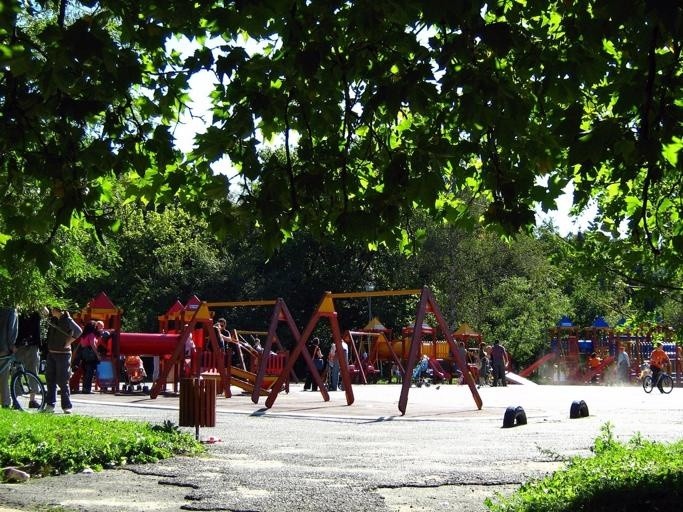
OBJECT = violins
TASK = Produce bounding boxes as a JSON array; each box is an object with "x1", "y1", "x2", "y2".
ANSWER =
[
  {"x1": 510, "y1": 352, "x2": 557, "y2": 384},
  {"x1": 228, "y1": 367, "x2": 278, "y2": 396},
  {"x1": 577, "y1": 356, "x2": 614, "y2": 385},
  {"x1": 426, "y1": 355, "x2": 452, "y2": 381}
]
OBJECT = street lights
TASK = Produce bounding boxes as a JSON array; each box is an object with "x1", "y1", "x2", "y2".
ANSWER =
[
  {"x1": 83, "y1": 348, "x2": 97, "y2": 360},
  {"x1": 314, "y1": 358, "x2": 323, "y2": 369}
]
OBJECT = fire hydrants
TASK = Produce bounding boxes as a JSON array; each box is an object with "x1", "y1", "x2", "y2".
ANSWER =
[{"x1": 356, "y1": 334, "x2": 376, "y2": 376}]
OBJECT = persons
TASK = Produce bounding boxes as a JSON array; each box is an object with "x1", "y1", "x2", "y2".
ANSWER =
[
  {"x1": 0, "y1": 301, "x2": 115, "y2": 414},
  {"x1": 302, "y1": 337, "x2": 509, "y2": 391},
  {"x1": 588, "y1": 343, "x2": 671, "y2": 393},
  {"x1": 183, "y1": 318, "x2": 264, "y2": 379}
]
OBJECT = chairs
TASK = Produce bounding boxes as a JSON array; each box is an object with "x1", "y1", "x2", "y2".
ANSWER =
[
  {"x1": 29, "y1": 400, "x2": 41, "y2": 407},
  {"x1": 63, "y1": 407, "x2": 73, "y2": 413},
  {"x1": 39, "y1": 406, "x2": 55, "y2": 413}
]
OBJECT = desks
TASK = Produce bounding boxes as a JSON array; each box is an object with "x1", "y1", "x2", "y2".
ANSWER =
[{"x1": 366, "y1": 281, "x2": 376, "y2": 348}]
